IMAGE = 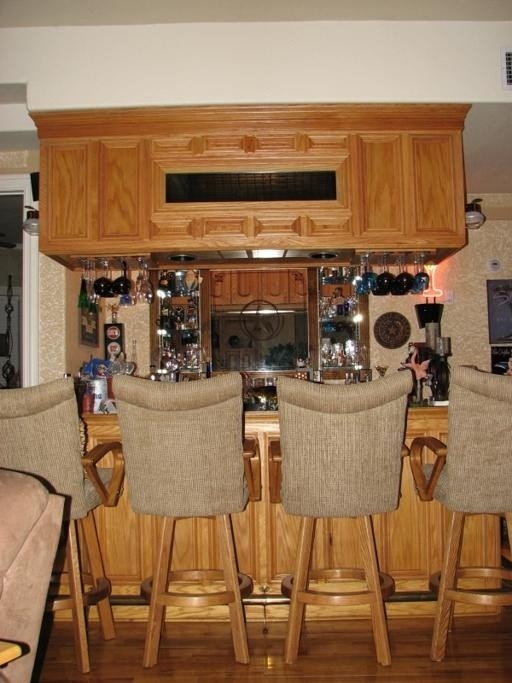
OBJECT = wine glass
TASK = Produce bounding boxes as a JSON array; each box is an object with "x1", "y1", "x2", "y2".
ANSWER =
[
  {"x1": 83, "y1": 257, "x2": 153, "y2": 314},
  {"x1": 357, "y1": 252, "x2": 430, "y2": 296}
]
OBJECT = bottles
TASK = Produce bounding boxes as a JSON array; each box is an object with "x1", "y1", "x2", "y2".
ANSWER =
[
  {"x1": 157, "y1": 269, "x2": 202, "y2": 374},
  {"x1": 314, "y1": 265, "x2": 365, "y2": 370}
]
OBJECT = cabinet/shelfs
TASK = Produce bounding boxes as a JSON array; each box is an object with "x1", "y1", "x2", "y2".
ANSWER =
[
  {"x1": 350, "y1": 125, "x2": 469, "y2": 249},
  {"x1": 39, "y1": 133, "x2": 148, "y2": 254}
]
